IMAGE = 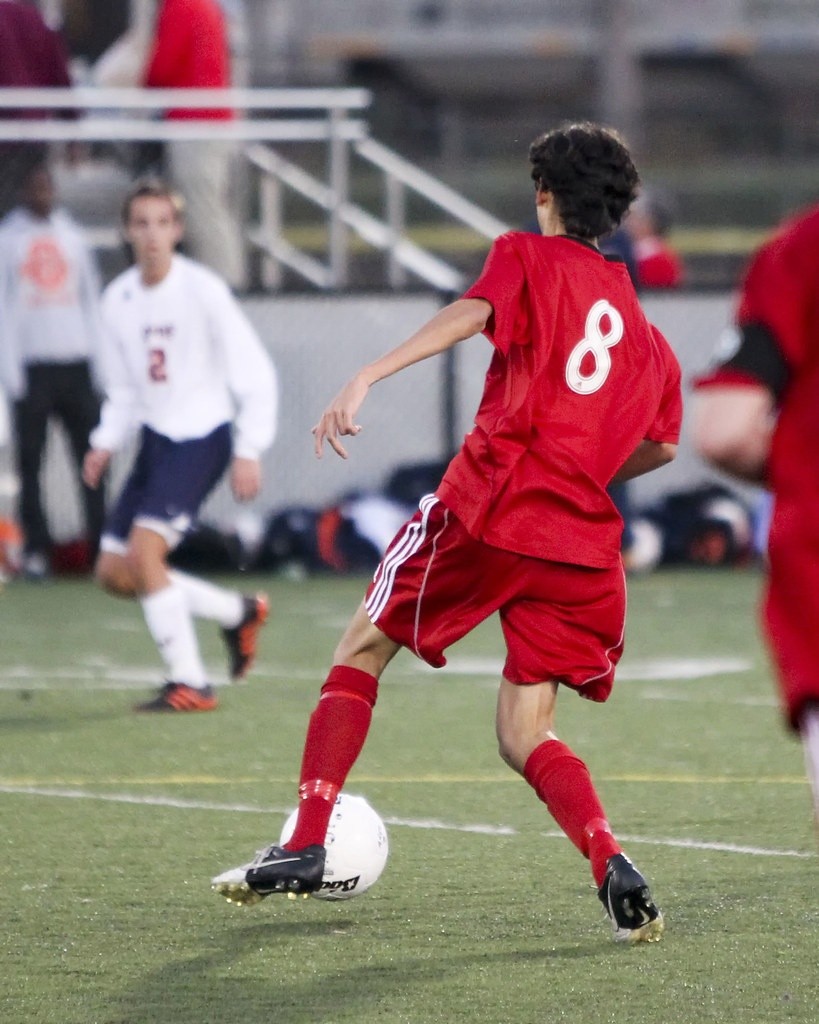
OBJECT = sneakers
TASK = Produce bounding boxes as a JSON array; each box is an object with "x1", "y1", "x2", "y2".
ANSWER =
[
  {"x1": 132, "y1": 681, "x2": 216, "y2": 715},
  {"x1": 210, "y1": 842, "x2": 326, "y2": 908},
  {"x1": 220, "y1": 596, "x2": 269, "y2": 680},
  {"x1": 595, "y1": 852, "x2": 664, "y2": 946}
]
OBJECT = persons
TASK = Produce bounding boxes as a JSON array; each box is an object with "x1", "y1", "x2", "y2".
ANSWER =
[
  {"x1": 0, "y1": 0, "x2": 280, "y2": 711},
  {"x1": 201, "y1": 124, "x2": 819, "y2": 945}
]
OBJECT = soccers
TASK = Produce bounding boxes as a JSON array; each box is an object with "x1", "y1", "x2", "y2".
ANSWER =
[{"x1": 280, "y1": 793, "x2": 388, "y2": 902}]
